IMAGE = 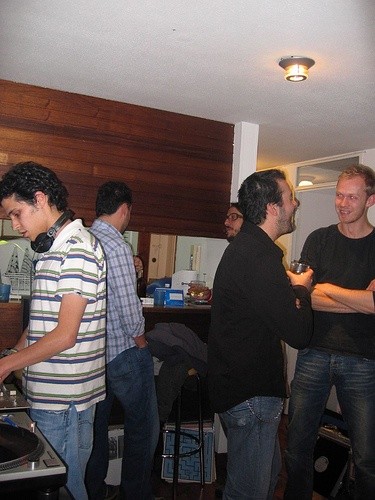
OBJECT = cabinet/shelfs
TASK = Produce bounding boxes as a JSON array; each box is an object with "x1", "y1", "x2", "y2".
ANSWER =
[{"x1": 137, "y1": 232, "x2": 178, "y2": 297}]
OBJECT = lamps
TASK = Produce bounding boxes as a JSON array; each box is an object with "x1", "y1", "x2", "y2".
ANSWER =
[{"x1": 279, "y1": 57, "x2": 315, "y2": 82}]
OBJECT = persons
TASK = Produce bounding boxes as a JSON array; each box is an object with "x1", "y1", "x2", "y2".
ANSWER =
[
  {"x1": 223, "y1": 203, "x2": 244, "y2": 244},
  {"x1": 84, "y1": 182, "x2": 160, "y2": 500},
  {"x1": 283, "y1": 163, "x2": 375, "y2": 500},
  {"x1": 0, "y1": 162, "x2": 106, "y2": 500},
  {"x1": 209, "y1": 168, "x2": 313, "y2": 500}
]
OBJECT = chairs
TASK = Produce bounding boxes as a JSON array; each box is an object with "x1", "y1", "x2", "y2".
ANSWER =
[{"x1": 147, "y1": 323, "x2": 210, "y2": 500}]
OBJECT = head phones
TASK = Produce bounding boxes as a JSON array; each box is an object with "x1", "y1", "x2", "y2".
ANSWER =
[{"x1": 31, "y1": 209, "x2": 76, "y2": 253}]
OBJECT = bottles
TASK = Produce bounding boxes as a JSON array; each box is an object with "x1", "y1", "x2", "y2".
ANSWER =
[{"x1": 137, "y1": 270, "x2": 147, "y2": 301}]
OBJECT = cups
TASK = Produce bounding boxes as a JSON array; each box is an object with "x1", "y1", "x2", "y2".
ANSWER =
[
  {"x1": 0, "y1": 283, "x2": 10, "y2": 302},
  {"x1": 154, "y1": 292, "x2": 165, "y2": 306},
  {"x1": 289, "y1": 263, "x2": 309, "y2": 275},
  {"x1": 191, "y1": 281, "x2": 206, "y2": 297}
]
